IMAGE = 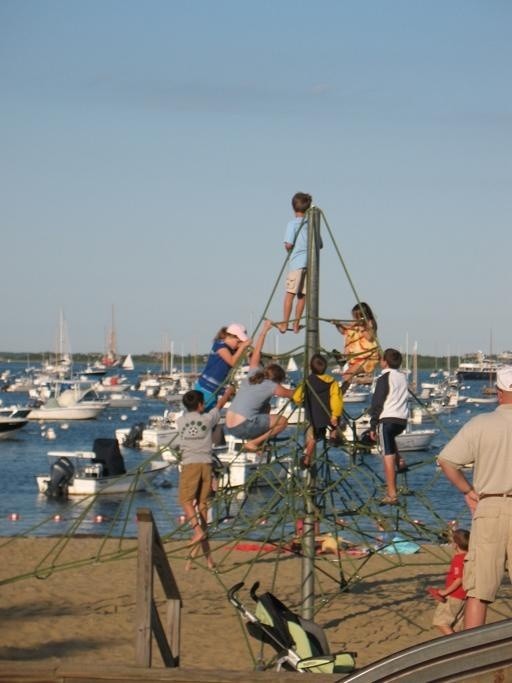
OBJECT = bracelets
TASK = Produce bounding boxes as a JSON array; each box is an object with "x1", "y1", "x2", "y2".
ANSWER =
[{"x1": 462, "y1": 486, "x2": 472, "y2": 495}]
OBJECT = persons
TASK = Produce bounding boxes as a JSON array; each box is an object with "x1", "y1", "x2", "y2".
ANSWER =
[
  {"x1": 369, "y1": 349, "x2": 409, "y2": 504},
  {"x1": 226, "y1": 322, "x2": 298, "y2": 451},
  {"x1": 293, "y1": 355, "x2": 344, "y2": 466},
  {"x1": 430, "y1": 528, "x2": 470, "y2": 637},
  {"x1": 186, "y1": 473, "x2": 221, "y2": 570},
  {"x1": 436, "y1": 365, "x2": 511, "y2": 633},
  {"x1": 330, "y1": 300, "x2": 378, "y2": 385},
  {"x1": 274, "y1": 191, "x2": 324, "y2": 333},
  {"x1": 195, "y1": 324, "x2": 252, "y2": 416},
  {"x1": 177, "y1": 387, "x2": 232, "y2": 546}
]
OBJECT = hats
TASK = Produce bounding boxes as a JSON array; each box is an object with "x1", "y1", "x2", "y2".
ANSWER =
[
  {"x1": 495, "y1": 368, "x2": 512, "y2": 391},
  {"x1": 225, "y1": 323, "x2": 249, "y2": 342}
]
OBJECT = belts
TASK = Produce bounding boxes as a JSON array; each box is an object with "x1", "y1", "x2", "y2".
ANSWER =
[{"x1": 479, "y1": 492, "x2": 512, "y2": 500}]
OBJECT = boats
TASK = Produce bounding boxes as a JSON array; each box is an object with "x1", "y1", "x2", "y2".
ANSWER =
[
  {"x1": 213, "y1": 436, "x2": 293, "y2": 486},
  {"x1": 227, "y1": 319, "x2": 512, "y2": 453},
  {"x1": 0, "y1": 405, "x2": 30, "y2": 437},
  {"x1": 116, "y1": 409, "x2": 182, "y2": 452},
  {"x1": 1, "y1": 300, "x2": 200, "y2": 420},
  {"x1": 33, "y1": 438, "x2": 170, "y2": 495}
]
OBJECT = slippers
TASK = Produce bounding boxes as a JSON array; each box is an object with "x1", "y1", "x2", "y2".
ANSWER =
[{"x1": 429, "y1": 588, "x2": 446, "y2": 601}]
